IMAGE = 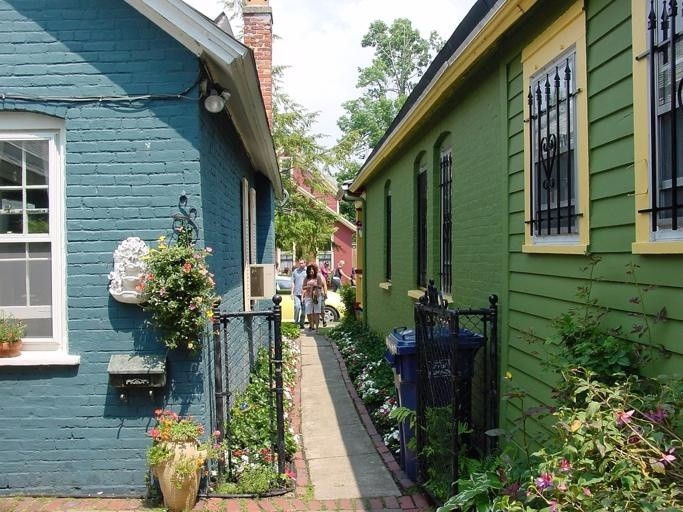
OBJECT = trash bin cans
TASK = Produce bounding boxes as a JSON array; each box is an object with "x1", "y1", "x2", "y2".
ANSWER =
[{"x1": 384, "y1": 325, "x2": 488, "y2": 482}]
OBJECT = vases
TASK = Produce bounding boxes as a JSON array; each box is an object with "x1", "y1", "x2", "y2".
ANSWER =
[{"x1": 152, "y1": 441, "x2": 202, "y2": 512}]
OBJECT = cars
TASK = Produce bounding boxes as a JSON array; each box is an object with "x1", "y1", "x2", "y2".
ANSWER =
[{"x1": 273, "y1": 272, "x2": 347, "y2": 326}]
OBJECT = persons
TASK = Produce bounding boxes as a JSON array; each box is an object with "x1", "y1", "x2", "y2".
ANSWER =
[
  {"x1": 320, "y1": 262, "x2": 330, "y2": 283},
  {"x1": 282, "y1": 266, "x2": 289, "y2": 275},
  {"x1": 275, "y1": 260, "x2": 279, "y2": 285},
  {"x1": 300, "y1": 263, "x2": 322, "y2": 334},
  {"x1": 314, "y1": 265, "x2": 327, "y2": 327},
  {"x1": 290, "y1": 258, "x2": 308, "y2": 328},
  {"x1": 332, "y1": 260, "x2": 351, "y2": 292}
]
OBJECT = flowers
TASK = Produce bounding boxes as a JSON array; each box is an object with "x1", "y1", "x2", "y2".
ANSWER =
[
  {"x1": 145, "y1": 407, "x2": 223, "y2": 490},
  {"x1": 133, "y1": 226, "x2": 221, "y2": 354}
]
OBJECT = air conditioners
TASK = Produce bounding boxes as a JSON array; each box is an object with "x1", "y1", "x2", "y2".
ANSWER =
[{"x1": 249, "y1": 263, "x2": 277, "y2": 300}]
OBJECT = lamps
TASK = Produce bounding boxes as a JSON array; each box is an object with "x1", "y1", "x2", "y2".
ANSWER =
[{"x1": 200, "y1": 77, "x2": 232, "y2": 113}]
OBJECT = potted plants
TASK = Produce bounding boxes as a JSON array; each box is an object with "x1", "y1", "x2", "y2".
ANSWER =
[{"x1": 0, "y1": 311, "x2": 27, "y2": 358}]
